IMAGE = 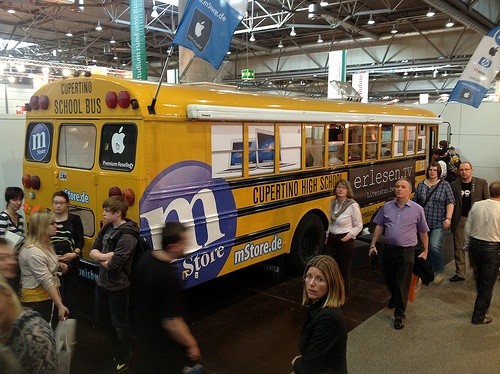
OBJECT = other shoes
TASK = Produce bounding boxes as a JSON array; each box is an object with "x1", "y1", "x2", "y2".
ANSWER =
[
  {"x1": 110, "y1": 361, "x2": 134, "y2": 374},
  {"x1": 432, "y1": 273, "x2": 442, "y2": 285},
  {"x1": 479, "y1": 317, "x2": 492, "y2": 325}
]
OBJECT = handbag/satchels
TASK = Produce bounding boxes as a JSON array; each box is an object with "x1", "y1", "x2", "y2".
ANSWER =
[{"x1": 53, "y1": 318, "x2": 77, "y2": 374}]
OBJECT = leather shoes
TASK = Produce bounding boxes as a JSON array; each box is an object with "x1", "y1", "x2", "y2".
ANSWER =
[
  {"x1": 449, "y1": 275, "x2": 464, "y2": 283},
  {"x1": 394, "y1": 315, "x2": 405, "y2": 329},
  {"x1": 387, "y1": 297, "x2": 396, "y2": 309}
]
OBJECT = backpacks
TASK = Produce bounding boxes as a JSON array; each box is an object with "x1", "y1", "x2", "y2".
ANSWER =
[{"x1": 100, "y1": 226, "x2": 152, "y2": 262}]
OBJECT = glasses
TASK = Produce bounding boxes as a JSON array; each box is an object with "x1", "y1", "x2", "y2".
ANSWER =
[
  {"x1": 303, "y1": 274, "x2": 327, "y2": 284},
  {"x1": 52, "y1": 201, "x2": 65, "y2": 206},
  {"x1": 48, "y1": 221, "x2": 56, "y2": 225}
]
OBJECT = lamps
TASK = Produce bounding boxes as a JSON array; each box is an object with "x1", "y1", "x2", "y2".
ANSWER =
[
  {"x1": 66, "y1": 20, "x2": 118, "y2": 59},
  {"x1": 307, "y1": 3, "x2": 319, "y2": 20},
  {"x1": 317, "y1": 35, "x2": 324, "y2": 44},
  {"x1": 367, "y1": 9, "x2": 454, "y2": 34},
  {"x1": 289, "y1": 25, "x2": 297, "y2": 37},
  {"x1": 278, "y1": 39, "x2": 285, "y2": 48},
  {"x1": 150, "y1": 0, "x2": 159, "y2": 17},
  {"x1": 249, "y1": 33, "x2": 255, "y2": 42}
]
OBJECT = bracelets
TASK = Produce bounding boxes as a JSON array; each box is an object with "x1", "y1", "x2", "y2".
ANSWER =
[
  {"x1": 74, "y1": 251, "x2": 78, "y2": 255},
  {"x1": 369, "y1": 246, "x2": 375, "y2": 250},
  {"x1": 446, "y1": 218, "x2": 451, "y2": 219}
]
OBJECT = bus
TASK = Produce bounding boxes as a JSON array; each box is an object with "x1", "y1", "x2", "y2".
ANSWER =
[{"x1": 22, "y1": 70, "x2": 453, "y2": 323}]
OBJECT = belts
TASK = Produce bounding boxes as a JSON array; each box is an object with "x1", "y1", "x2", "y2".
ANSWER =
[
  {"x1": 470, "y1": 239, "x2": 498, "y2": 246},
  {"x1": 383, "y1": 243, "x2": 415, "y2": 251}
]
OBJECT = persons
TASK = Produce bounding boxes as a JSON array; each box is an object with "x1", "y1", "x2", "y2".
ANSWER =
[
  {"x1": 0, "y1": 238, "x2": 23, "y2": 331},
  {"x1": 49, "y1": 191, "x2": 84, "y2": 328},
  {"x1": 172, "y1": 0, "x2": 249, "y2": 70},
  {"x1": 413, "y1": 162, "x2": 457, "y2": 284},
  {"x1": 291, "y1": 255, "x2": 348, "y2": 374},
  {"x1": 0, "y1": 185, "x2": 27, "y2": 254},
  {"x1": 324, "y1": 179, "x2": 363, "y2": 297},
  {"x1": 450, "y1": 163, "x2": 490, "y2": 281},
  {"x1": 0, "y1": 310, "x2": 58, "y2": 374},
  {"x1": 89, "y1": 195, "x2": 141, "y2": 374},
  {"x1": 326, "y1": 123, "x2": 392, "y2": 165},
  {"x1": 18, "y1": 211, "x2": 69, "y2": 322},
  {"x1": 432, "y1": 140, "x2": 461, "y2": 183},
  {"x1": 461, "y1": 181, "x2": 500, "y2": 324},
  {"x1": 369, "y1": 179, "x2": 430, "y2": 329},
  {"x1": 447, "y1": 24, "x2": 500, "y2": 108},
  {"x1": 129, "y1": 222, "x2": 200, "y2": 374}
]
